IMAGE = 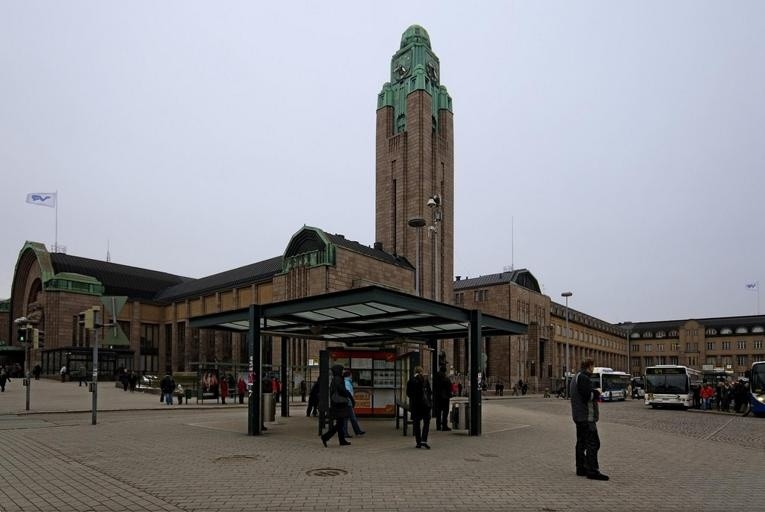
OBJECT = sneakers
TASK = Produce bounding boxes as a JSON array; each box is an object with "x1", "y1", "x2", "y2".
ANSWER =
[
  {"x1": 437, "y1": 427, "x2": 452, "y2": 432},
  {"x1": 415, "y1": 441, "x2": 431, "y2": 450},
  {"x1": 320, "y1": 434, "x2": 329, "y2": 448},
  {"x1": 344, "y1": 434, "x2": 353, "y2": 438},
  {"x1": 575, "y1": 466, "x2": 610, "y2": 481},
  {"x1": 353, "y1": 432, "x2": 366, "y2": 436},
  {"x1": 338, "y1": 441, "x2": 352, "y2": 446}
]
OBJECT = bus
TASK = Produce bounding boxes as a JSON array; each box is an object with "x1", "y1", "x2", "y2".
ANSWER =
[
  {"x1": 644, "y1": 364, "x2": 704, "y2": 408},
  {"x1": 745, "y1": 361, "x2": 765, "y2": 414},
  {"x1": 631, "y1": 376, "x2": 645, "y2": 398},
  {"x1": 590, "y1": 365, "x2": 631, "y2": 401}
]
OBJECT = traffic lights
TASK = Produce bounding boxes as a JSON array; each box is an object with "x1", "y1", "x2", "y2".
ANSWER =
[
  {"x1": 79, "y1": 309, "x2": 89, "y2": 328},
  {"x1": 34, "y1": 329, "x2": 44, "y2": 350},
  {"x1": 18, "y1": 327, "x2": 25, "y2": 342}
]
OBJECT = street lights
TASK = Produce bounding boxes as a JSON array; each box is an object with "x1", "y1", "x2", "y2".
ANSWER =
[
  {"x1": 561, "y1": 292, "x2": 572, "y2": 400},
  {"x1": 427, "y1": 196, "x2": 441, "y2": 373},
  {"x1": 408, "y1": 215, "x2": 426, "y2": 369},
  {"x1": 13, "y1": 316, "x2": 28, "y2": 386}
]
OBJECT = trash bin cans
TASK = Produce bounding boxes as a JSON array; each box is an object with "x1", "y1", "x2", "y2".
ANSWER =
[
  {"x1": 450, "y1": 397, "x2": 469, "y2": 434},
  {"x1": 262, "y1": 393, "x2": 275, "y2": 422}
]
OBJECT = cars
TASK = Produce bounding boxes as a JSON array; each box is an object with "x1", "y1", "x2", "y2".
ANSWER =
[{"x1": 139, "y1": 374, "x2": 160, "y2": 385}]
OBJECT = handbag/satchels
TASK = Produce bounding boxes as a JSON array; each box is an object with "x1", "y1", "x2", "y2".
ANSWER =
[
  {"x1": 422, "y1": 379, "x2": 435, "y2": 409},
  {"x1": 331, "y1": 392, "x2": 350, "y2": 404}
]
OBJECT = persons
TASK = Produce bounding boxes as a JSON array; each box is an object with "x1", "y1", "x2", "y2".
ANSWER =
[
  {"x1": 406, "y1": 366, "x2": 433, "y2": 449},
  {"x1": 129, "y1": 371, "x2": 138, "y2": 392},
  {"x1": 570, "y1": 357, "x2": 610, "y2": 481},
  {"x1": 321, "y1": 364, "x2": 356, "y2": 448},
  {"x1": 452, "y1": 377, "x2": 528, "y2": 396},
  {"x1": 174, "y1": 384, "x2": 185, "y2": 405},
  {"x1": 121, "y1": 368, "x2": 130, "y2": 392},
  {"x1": 434, "y1": 367, "x2": 452, "y2": 432},
  {"x1": 60, "y1": 364, "x2": 67, "y2": 383},
  {"x1": 343, "y1": 371, "x2": 366, "y2": 438},
  {"x1": 79, "y1": 364, "x2": 88, "y2": 387},
  {"x1": 220, "y1": 373, "x2": 320, "y2": 417},
  {"x1": 33, "y1": 363, "x2": 41, "y2": 380},
  {"x1": 0, "y1": 363, "x2": 22, "y2": 392},
  {"x1": 160, "y1": 371, "x2": 176, "y2": 405},
  {"x1": 691, "y1": 377, "x2": 749, "y2": 413}
]
